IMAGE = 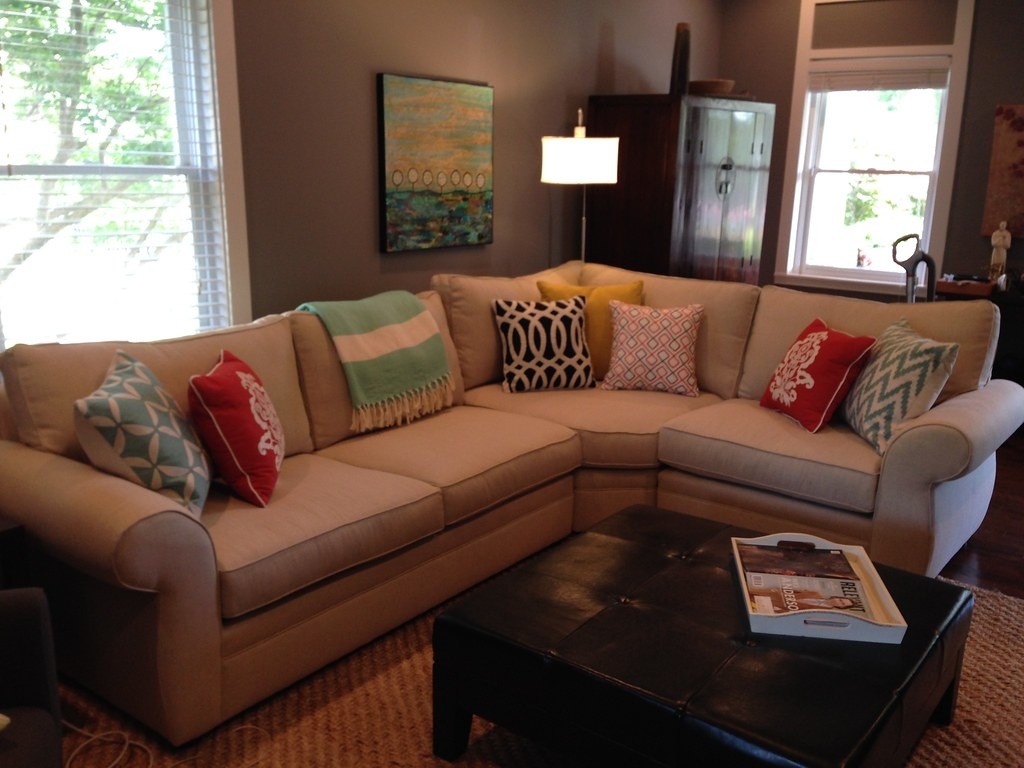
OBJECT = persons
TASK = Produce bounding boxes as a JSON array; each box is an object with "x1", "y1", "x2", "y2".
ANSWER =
[
  {"x1": 989, "y1": 220, "x2": 1012, "y2": 280},
  {"x1": 747, "y1": 585, "x2": 855, "y2": 617}
]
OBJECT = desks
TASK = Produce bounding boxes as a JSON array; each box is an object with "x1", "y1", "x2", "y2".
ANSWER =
[{"x1": 937, "y1": 278, "x2": 997, "y2": 298}]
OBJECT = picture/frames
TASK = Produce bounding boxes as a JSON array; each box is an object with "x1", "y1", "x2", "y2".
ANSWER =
[{"x1": 377, "y1": 72, "x2": 496, "y2": 253}]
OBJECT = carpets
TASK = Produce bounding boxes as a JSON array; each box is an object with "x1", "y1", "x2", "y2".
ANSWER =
[{"x1": 65, "y1": 573, "x2": 1024, "y2": 768}]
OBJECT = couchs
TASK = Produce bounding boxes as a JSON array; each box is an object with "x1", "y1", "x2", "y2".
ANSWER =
[{"x1": 0, "y1": 257, "x2": 1024, "y2": 752}]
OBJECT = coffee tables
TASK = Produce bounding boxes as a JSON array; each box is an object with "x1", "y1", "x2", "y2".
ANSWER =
[{"x1": 428, "y1": 502, "x2": 978, "y2": 768}]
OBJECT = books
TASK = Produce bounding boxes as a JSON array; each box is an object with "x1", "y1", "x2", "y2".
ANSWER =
[
  {"x1": 746, "y1": 571, "x2": 877, "y2": 626},
  {"x1": 738, "y1": 542, "x2": 858, "y2": 580}
]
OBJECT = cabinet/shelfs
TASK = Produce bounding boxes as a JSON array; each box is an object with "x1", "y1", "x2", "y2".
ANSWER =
[{"x1": 580, "y1": 91, "x2": 778, "y2": 285}]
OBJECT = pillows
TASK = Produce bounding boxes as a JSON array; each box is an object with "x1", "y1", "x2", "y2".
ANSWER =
[
  {"x1": 489, "y1": 294, "x2": 597, "y2": 391},
  {"x1": 599, "y1": 300, "x2": 706, "y2": 400},
  {"x1": 842, "y1": 317, "x2": 963, "y2": 453},
  {"x1": 188, "y1": 349, "x2": 288, "y2": 510},
  {"x1": 760, "y1": 317, "x2": 878, "y2": 435},
  {"x1": 73, "y1": 347, "x2": 212, "y2": 524},
  {"x1": 536, "y1": 280, "x2": 645, "y2": 380}
]
